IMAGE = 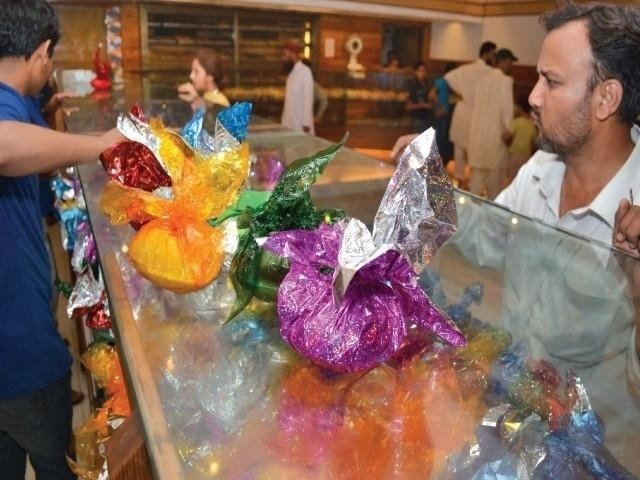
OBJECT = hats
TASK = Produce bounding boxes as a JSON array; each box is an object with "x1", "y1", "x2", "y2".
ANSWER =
[
  {"x1": 495, "y1": 49, "x2": 518, "y2": 61},
  {"x1": 282, "y1": 37, "x2": 301, "y2": 53}
]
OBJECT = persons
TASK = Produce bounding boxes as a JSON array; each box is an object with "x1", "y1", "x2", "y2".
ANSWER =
[
  {"x1": 426, "y1": 62, "x2": 460, "y2": 162},
  {"x1": 443, "y1": 35, "x2": 498, "y2": 190},
  {"x1": 0, "y1": 1, "x2": 130, "y2": 480},
  {"x1": 465, "y1": 45, "x2": 520, "y2": 198},
  {"x1": 36, "y1": 81, "x2": 76, "y2": 226},
  {"x1": 384, "y1": 50, "x2": 405, "y2": 70},
  {"x1": 387, "y1": 0, "x2": 640, "y2": 479},
  {"x1": 404, "y1": 60, "x2": 430, "y2": 137},
  {"x1": 177, "y1": 48, "x2": 230, "y2": 111},
  {"x1": 501, "y1": 98, "x2": 537, "y2": 180},
  {"x1": 280, "y1": 38, "x2": 316, "y2": 173}
]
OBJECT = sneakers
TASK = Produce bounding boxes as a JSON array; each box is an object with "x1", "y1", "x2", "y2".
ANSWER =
[{"x1": 45, "y1": 216, "x2": 58, "y2": 226}]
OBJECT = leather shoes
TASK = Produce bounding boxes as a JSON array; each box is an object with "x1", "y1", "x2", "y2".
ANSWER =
[{"x1": 70, "y1": 389, "x2": 84, "y2": 405}]
